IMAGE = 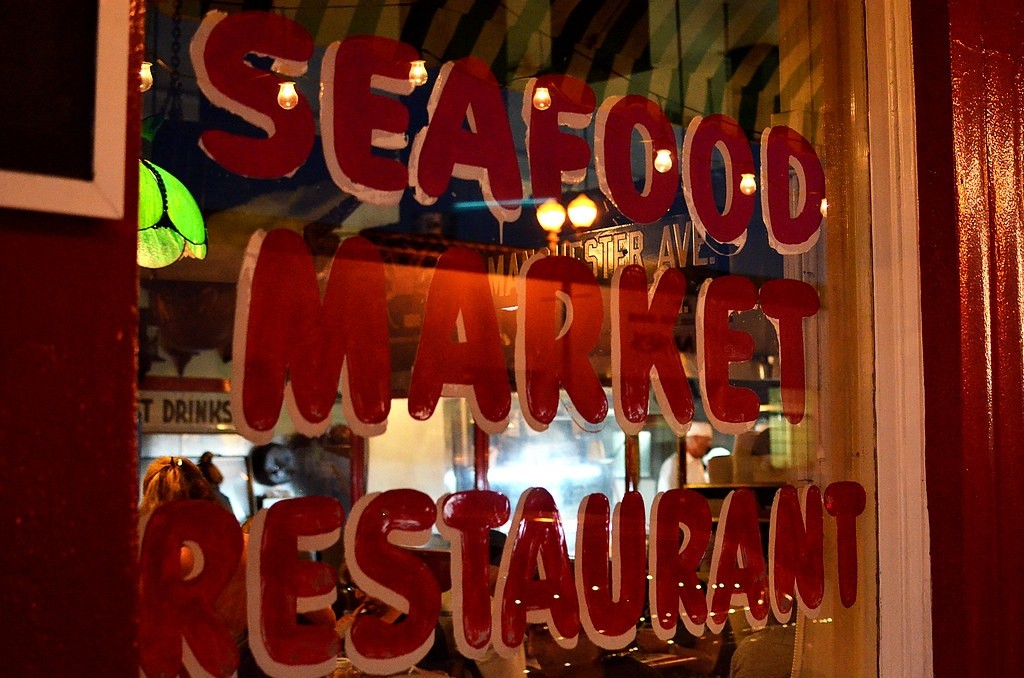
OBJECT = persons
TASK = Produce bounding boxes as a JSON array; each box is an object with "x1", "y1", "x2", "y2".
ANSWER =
[
  {"x1": 136, "y1": 453, "x2": 736, "y2": 678},
  {"x1": 656, "y1": 420, "x2": 712, "y2": 493}
]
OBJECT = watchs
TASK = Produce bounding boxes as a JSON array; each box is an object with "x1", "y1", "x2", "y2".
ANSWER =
[{"x1": 666, "y1": 640, "x2": 678, "y2": 655}]
